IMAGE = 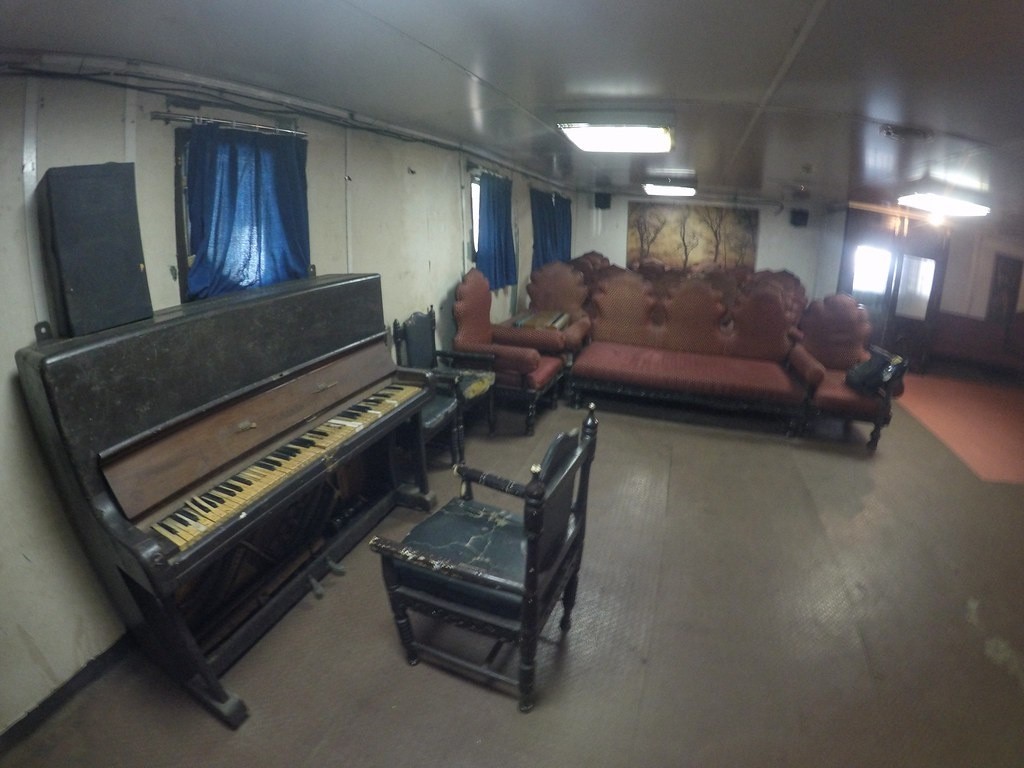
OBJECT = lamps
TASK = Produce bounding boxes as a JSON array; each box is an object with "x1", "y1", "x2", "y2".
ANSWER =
[
  {"x1": 897, "y1": 176, "x2": 991, "y2": 217},
  {"x1": 554, "y1": 110, "x2": 675, "y2": 153},
  {"x1": 639, "y1": 174, "x2": 698, "y2": 197}
]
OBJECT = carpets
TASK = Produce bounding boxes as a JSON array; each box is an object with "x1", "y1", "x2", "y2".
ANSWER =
[{"x1": 893, "y1": 373, "x2": 1024, "y2": 483}]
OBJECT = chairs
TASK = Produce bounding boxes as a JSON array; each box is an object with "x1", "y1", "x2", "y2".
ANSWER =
[
  {"x1": 392, "y1": 304, "x2": 499, "y2": 466},
  {"x1": 797, "y1": 291, "x2": 895, "y2": 450},
  {"x1": 450, "y1": 250, "x2": 623, "y2": 435},
  {"x1": 384, "y1": 327, "x2": 460, "y2": 480},
  {"x1": 370, "y1": 402, "x2": 598, "y2": 711}
]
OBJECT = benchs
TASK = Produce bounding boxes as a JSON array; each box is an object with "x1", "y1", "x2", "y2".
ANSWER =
[{"x1": 570, "y1": 260, "x2": 805, "y2": 436}]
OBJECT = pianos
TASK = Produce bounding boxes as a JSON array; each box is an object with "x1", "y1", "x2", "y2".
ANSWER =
[{"x1": 13, "y1": 267, "x2": 439, "y2": 734}]
